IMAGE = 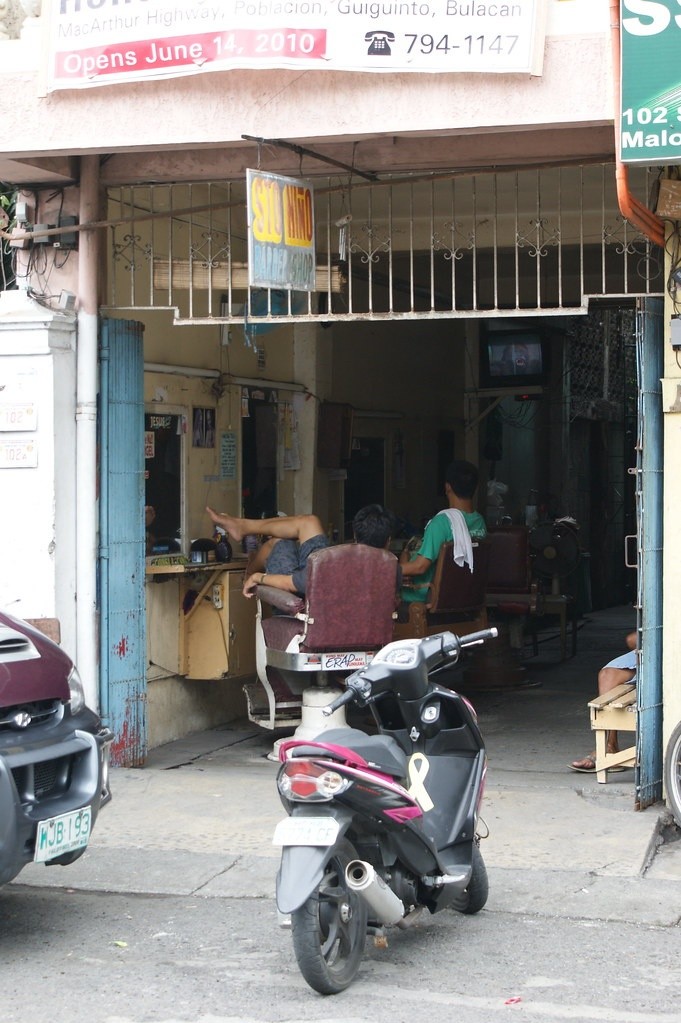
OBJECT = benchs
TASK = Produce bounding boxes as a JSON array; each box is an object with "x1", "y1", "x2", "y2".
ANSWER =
[{"x1": 588, "y1": 682, "x2": 639, "y2": 784}]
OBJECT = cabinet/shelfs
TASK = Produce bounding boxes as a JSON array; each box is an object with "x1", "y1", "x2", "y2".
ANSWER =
[{"x1": 155, "y1": 571, "x2": 271, "y2": 679}]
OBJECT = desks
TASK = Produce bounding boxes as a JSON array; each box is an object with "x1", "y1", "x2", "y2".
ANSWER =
[{"x1": 526, "y1": 592, "x2": 578, "y2": 659}]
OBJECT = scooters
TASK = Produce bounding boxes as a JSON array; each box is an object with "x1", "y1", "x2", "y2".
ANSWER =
[{"x1": 276, "y1": 627, "x2": 501, "y2": 996}]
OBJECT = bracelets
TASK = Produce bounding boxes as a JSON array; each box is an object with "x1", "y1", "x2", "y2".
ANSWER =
[{"x1": 262, "y1": 574, "x2": 266, "y2": 582}]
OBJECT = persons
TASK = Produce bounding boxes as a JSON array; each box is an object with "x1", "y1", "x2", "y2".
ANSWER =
[
  {"x1": 399, "y1": 460, "x2": 488, "y2": 601},
  {"x1": 567, "y1": 633, "x2": 637, "y2": 772},
  {"x1": 206, "y1": 503, "x2": 403, "y2": 615}
]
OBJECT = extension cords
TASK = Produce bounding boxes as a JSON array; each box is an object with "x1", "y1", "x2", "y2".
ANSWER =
[{"x1": 213, "y1": 584, "x2": 222, "y2": 609}]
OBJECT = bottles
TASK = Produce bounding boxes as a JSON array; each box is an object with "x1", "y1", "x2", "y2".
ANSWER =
[
  {"x1": 245, "y1": 534, "x2": 257, "y2": 557},
  {"x1": 525, "y1": 488, "x2": 539, "y2": 527},
  {"x1": 327, "y1": 522, "x2": 333, "y2": 546}
]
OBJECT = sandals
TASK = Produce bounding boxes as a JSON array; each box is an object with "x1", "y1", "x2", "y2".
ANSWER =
[{"x1": 567, "y1": 744, "x2": 625, "y2": 772}]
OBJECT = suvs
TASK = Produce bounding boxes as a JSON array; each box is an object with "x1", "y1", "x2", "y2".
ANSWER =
[{"x1": 0, "y1": 610, "x2": 117, "y2": 887}]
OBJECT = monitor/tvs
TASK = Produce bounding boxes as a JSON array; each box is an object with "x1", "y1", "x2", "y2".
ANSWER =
[{"x1": 478, "y1": 328, "x2": 551, "y2": 388}]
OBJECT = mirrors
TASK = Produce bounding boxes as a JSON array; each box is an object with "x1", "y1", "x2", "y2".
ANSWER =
[
  {"x1": 142, "y1": 403, "x2": 190, "y2": 561},
  {"x1": 238, "y1": 386, "x2": 281, "y2": 557},
  {"x1": 340, "y1": 433, "x2": 394, "y2": 543}
]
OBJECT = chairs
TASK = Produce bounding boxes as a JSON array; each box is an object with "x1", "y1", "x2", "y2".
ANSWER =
[
  {"x1": 242, "y1": 545, "x2": 398, "y2": 762},
  {"x1": 393, "y1": 539, "x2": 492, "y2": 677},
  {"x1": 480, "y1": 524, "x2": 542, "y2": 674}
]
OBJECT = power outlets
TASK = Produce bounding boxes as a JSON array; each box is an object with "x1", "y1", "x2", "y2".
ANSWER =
[{"x1": 212, "y1": 584, "x2": 223, "y2": 609}]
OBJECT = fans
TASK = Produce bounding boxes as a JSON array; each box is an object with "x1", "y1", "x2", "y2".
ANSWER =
[{"x1": 527, "y1": 520, "x2": 580, "y2": 600}]
